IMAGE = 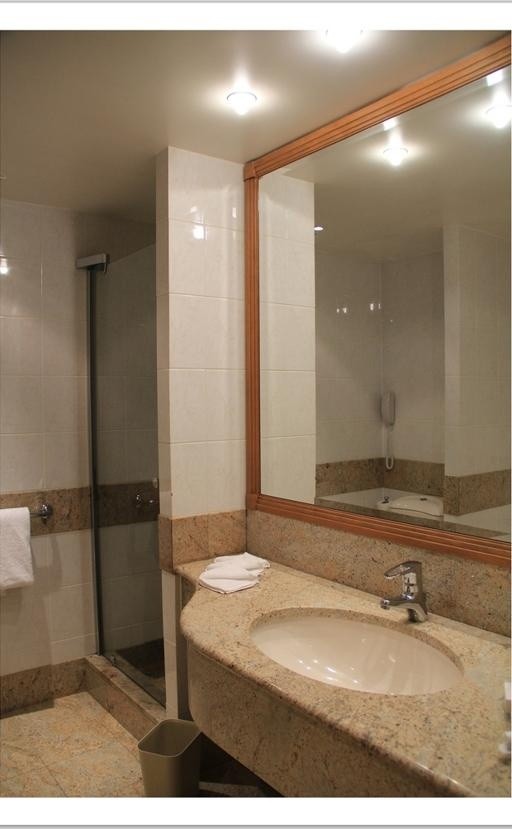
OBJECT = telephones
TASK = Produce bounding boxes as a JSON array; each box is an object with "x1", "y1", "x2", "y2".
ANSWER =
[{"x1": 380, "y1": 391, "x2": 396, "y2": 426}]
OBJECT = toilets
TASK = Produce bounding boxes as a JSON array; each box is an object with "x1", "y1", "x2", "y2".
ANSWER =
[{"x1": 388, "y1": 495, "x2": 443, "y2": 520}]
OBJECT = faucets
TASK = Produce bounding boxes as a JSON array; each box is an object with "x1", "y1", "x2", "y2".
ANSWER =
[{"x1": 380, "y1": 560, "x2": 427, "y2": 624}]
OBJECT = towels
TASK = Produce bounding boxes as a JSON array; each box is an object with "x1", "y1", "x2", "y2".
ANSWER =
[
  {"x1": 2, "y1": 502, "x2": 34, "y2": 599},
  {"x1": 197, "y1": 552, "x2": 270, "y2": 603}
]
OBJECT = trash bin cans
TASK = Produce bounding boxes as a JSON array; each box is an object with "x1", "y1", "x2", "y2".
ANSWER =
[{"x1": 137, "y1": 719, "x2": 203, "y2": 797}]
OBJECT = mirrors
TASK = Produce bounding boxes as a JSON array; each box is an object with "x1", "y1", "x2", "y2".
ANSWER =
[{"x1": 247, "y1": 32, "x2": 511, "y2": 576}]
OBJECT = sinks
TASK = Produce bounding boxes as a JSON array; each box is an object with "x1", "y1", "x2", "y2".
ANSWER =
[{"x1": 250, "y1": 606, "x2": 464, "y2": 694}]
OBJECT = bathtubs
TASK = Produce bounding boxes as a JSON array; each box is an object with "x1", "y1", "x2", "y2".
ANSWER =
[{"x1": 319, "y1": 488, "x2": 421, "y2": 510}]
